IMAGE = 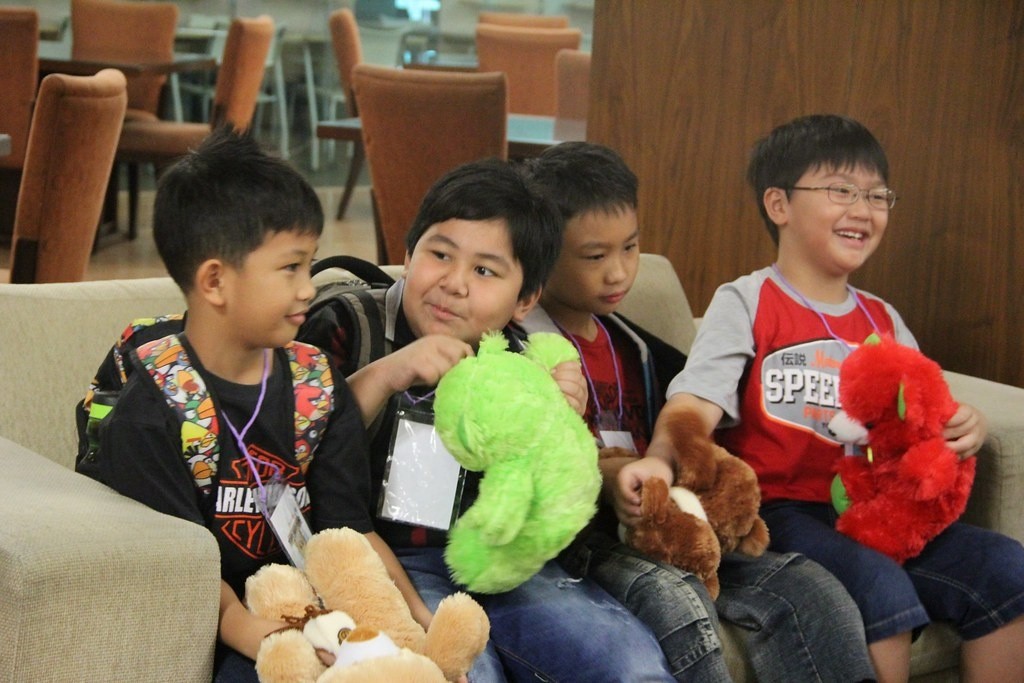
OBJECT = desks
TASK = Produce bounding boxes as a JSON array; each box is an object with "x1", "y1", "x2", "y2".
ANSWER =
[
  {"x1": 315, "y1": 112, "x2": 584, "y2": 161},
  {"x1": 34, "y1": 39, "x2": 217, "y2": 125}
]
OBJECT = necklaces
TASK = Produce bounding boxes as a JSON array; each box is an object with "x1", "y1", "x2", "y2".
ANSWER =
[{"x1": 772, "y1": 262, "x2": 879, "y2": 351}]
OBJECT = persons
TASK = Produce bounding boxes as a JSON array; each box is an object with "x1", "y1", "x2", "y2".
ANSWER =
[
  {"x1": 520, "y1": 140, "x2": 881, "y2": 683},
  {"x1": 291, "y1": 156, "x2": 680, "y2": 683},
  {"x1": 96, "y1": 124, "x2": 471, "y2": 683},
  {"x1": 614, "y1": 114, "x2": 1024, "y2": 683}
]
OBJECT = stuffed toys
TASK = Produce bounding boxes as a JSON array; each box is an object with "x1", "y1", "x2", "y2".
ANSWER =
[
  {"x1": 620, "y1": 407, "x2": 771, "y2": 601},
  {"x1": 245, "y1": 526, "x2": 491, "y2": 683},
  {"x1": 432, "y1": 331, "x2": 603, "y2": 594},
  {"x1": 828, "y1": 331, "x2": 976, "y2": 566}
]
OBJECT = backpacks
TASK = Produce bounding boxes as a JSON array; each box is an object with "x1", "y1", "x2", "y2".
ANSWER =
[
  {"x1": 308, "y1": 256, "x2": 398, "y2": 445},
  {"x1": 74, "y1": 313, "x2": 335, "y2": 500}
]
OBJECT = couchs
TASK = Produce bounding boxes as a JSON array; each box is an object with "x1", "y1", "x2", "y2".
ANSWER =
[{"x1": 0, "y1": 253, "x2": 1024, "y2": 683}]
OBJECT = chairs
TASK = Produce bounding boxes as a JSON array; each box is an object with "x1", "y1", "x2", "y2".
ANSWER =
[
  {"x1": 475, "y1": 23, "x2": 582, "y2": 116},
  {"x1": 0, "y1": 66, "x2": 127, "y2": 284},
  {"x1": 553, "y1": 50, "x2": 593, "y2": 119},
  {"x1": 350, "y1": 64, "x2": 509, "y2": 263},
  {"x1": 329, "y1": 8, "x2": 367, "y2": 220},
  {"x1": 174, "y1": 21, "x2": 477, "y2": 169},
  {"x1": 118, "y1": 15, "x2": 274, "y2": 242},
  {"x1": 71, "y1": 1, "x2": 181, "y2": 240},
  {"x1": 480, "y1": 12, "x2": 567, "y2": 31},
  {"x1": 1, "y1": 8, "x2": 39, "y2": 169}
]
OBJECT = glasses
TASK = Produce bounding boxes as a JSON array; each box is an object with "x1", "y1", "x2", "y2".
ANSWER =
[{"x1": 780, "y1": 182, "x2": 900, "y2": 211}]
